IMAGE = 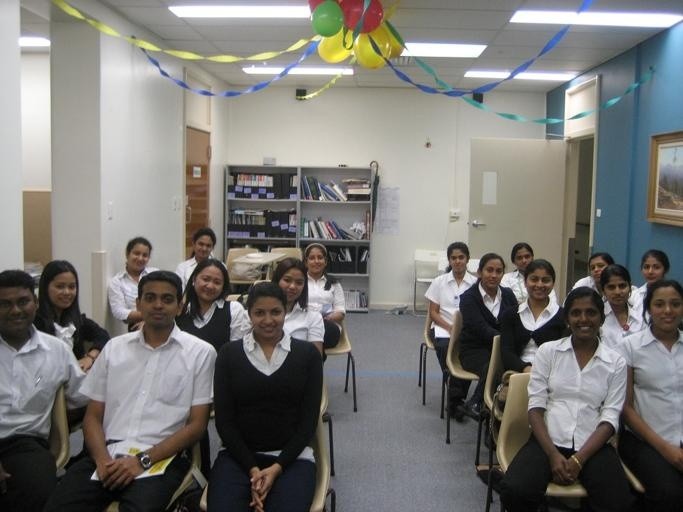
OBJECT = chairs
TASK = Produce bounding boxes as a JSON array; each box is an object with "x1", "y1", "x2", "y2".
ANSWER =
[
  {"x1": 106, "y1": 247, "x2": 357, "y2": 511},
  {"x1": 418, "y1": 305, "x2": 644, "y2": 512},
  {"x1": 413, "y1": 249, "x2": 438, "y2": 313},
  {"x1": 45, "y1": 384, "x2": 70, "y2": 472}
]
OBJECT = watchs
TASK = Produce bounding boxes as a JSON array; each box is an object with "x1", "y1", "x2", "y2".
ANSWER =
[{"x1": 136, "y1": 452, "x2": 151, "y2": 470}]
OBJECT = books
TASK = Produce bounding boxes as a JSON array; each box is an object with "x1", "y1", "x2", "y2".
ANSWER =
[{"x1": 302, "y1": 174, "x2": 370, "y2": 240}]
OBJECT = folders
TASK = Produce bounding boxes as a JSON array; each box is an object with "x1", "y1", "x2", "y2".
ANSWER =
[
  {"x1": 228, "y1": 207, "x2": 296, "y2": 238},
  {"x1": 228, "y1": 172, "x2": 297, "y2": 200}
]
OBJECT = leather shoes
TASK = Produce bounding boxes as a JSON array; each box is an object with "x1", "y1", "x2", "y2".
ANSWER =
[
  {"x1": 465, "y1": 398, "x2": 482, "y2": 420},
  {"x1": 484, "y1": 414, "x2": 497, "y2": 451}
]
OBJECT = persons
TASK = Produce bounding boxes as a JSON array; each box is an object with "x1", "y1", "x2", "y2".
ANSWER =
[
  {"x1": 621, "y1": 280, "x2": 683, "y2": 512},
  {"x1": 500, "y1": 286, "x2": 636, "y2": 512},
  {"x1": 0, "y1": 268, "x2": 90, "y2": 512},
  {"x1": 174, "y1": 258, "x2": 245, "y2": 356},
  {"x1": 302, "y1": 241, "x2": 346, "y2": 350},
  {"x1": 238, "y1": 257, "x2": 326, "y2": 359},
  {"x1": 172, "y1": 226, "x2": 227, "y2": 296},
  {"x1": 108, "y1": 236, "x2": 161, "y2": 333},
  {"x1": 46, "y1": 270, "x2": 218, "y2": 512},
  {"x1": 32, "y1": 259, "x2": 111, "y2": 435},
  {"x1": 422, "y1": 239, "x2": 670, "y2": 448},
  {"x1": 205, "y1": 281, "x2": 324, "y2": 512}
]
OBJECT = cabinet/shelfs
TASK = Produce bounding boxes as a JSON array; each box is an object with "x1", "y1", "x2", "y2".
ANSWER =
[{"x1": 225, "y1": 161, "x2": 373, "y2": 314}]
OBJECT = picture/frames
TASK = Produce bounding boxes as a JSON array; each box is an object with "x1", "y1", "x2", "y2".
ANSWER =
[{"x1": 646, "y1": 130, "x2": 682, "y2": 227}]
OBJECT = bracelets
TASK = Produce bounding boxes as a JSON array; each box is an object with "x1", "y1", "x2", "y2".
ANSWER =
[
  {"x1": 83, "y1": 353, "x2": 94, "y2": 360},
  {"x1": 571, "y1": 455, "x2": 582, "y2": 469}
]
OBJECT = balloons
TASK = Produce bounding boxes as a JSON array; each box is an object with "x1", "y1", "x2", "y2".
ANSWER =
[{"x1": 308, "y1": 0, "x2": 405, "y2": 70}]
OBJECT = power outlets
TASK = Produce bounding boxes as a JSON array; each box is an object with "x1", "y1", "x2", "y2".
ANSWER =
[{"x1": 449, "y1": 209, "x2": 460, "y2": 222}]
